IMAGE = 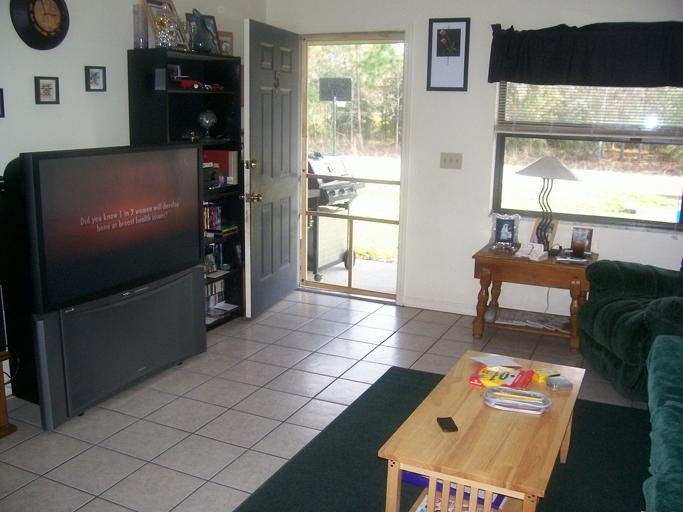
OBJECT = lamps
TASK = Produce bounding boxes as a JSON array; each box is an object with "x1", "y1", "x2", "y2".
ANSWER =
[{"x1": 516, "y1": 154, "x2": 578, "y2": 256}]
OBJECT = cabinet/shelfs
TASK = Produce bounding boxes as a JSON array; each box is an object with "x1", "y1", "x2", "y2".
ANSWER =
[
  {"x1": 128, "y1": 48, "x2": 245, "y2": 330},
  {"x1": 6, "y1": 264, "x2": 206, "y2": 433}
]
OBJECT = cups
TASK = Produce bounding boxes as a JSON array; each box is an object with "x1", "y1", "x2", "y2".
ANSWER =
[
  {"x1": 132, "y1": 3, "x2": 148, "y2": 50},
  {"x1": 571, "y1": 231, "x2": 587, "y2": 259}
]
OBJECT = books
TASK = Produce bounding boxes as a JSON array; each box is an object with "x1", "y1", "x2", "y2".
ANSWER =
[
  {"x1": 200, "y1": 196, "x2": 240, "y2": 324},
  {"x1": 415, "y1": 494, "x2": 485, "y2": 512},
  {"x1": 467, "y1": 363, "x2": 535, "y2": 393},
  {"x1": 401, "y1": 470, "x2": 508, "y2": 509}
]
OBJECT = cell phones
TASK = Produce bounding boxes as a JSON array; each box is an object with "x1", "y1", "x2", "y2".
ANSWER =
[{"x1": 437, "y1": 416, "x2": 458, "y2": 433}]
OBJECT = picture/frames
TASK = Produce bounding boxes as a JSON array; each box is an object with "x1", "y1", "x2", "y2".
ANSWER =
[
  {"x1": 489, "y1": 213, "x2": 559, "y2": 251},
  {"x1": 0, "y1": 66, "x2": 106, "y2": 117},
  {"x1": 426, "y1": 16, "x2": 470, "y2": 91},
  {"x1": 143, "y1": 0, "x2": 221, "y2": 54}
]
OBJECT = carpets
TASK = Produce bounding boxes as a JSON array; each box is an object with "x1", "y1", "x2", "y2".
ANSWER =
[{"x1": 231, "y1": 365, "x2": 651, "y2": 512}]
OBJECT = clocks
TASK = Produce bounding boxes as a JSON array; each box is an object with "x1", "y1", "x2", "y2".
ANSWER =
[{"x1": 10, "y1": 0, "x2": 69, "y2": 50}]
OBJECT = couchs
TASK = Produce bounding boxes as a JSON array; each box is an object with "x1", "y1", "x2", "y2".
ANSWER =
[{"x1": 576, "y1": 260, "x2": 683, "y2": 512}]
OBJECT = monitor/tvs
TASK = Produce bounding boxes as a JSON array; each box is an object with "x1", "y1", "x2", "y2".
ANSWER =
[{"x1": 31, "y1": 143, "x2": 205, "y2": 315}]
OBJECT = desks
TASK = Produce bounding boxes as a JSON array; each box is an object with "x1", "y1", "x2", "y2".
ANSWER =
[
  {"x1": 472, "y1": 242, "x2": 598, "y2": 353},
  {"x1": 378, "y1": 350, "x2": 585, "y2": 512}
]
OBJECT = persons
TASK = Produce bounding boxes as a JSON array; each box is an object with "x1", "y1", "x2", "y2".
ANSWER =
[{"x1": 498, "y1": 223, "x2": 511, "y2": 243}]
